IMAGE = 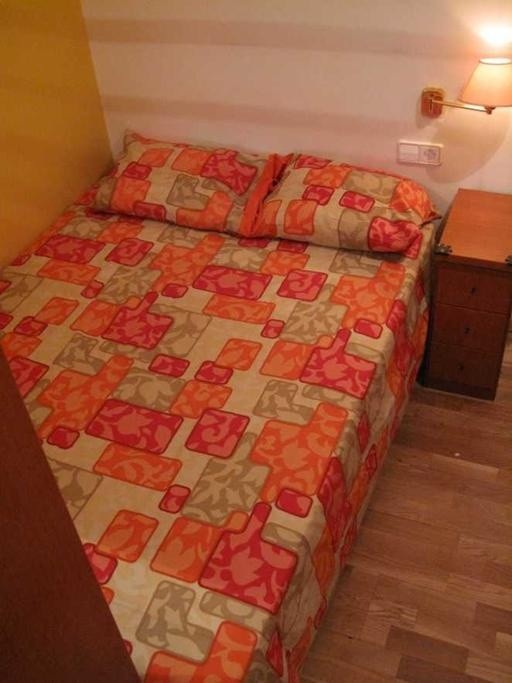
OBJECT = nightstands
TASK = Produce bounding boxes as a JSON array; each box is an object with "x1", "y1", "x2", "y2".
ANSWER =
[{"x1": 419, "y1": 188, "x2": 512, "y2": 401}]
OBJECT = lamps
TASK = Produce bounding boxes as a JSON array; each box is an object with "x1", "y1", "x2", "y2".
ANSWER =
[{"x1": 421, "y1": 56, "x2": 512, "y2": 119}]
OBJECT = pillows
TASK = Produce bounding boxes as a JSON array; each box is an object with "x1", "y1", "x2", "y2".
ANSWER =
[
  {"x1": 248, "y1": 153, "x2": 443, "y2": 260},
  {"x1": 80, "y1": 129, "x2": 286, "y2": 239}
]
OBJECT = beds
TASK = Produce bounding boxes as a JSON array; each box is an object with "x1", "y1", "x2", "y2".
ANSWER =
[{"x1": 1, "y1": 196, "x2": 439, "y2": 682}]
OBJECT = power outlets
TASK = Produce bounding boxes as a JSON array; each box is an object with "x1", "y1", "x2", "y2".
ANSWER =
[{"x1": 397, "y1": 140, "x2": 443, "y2": 167}]
